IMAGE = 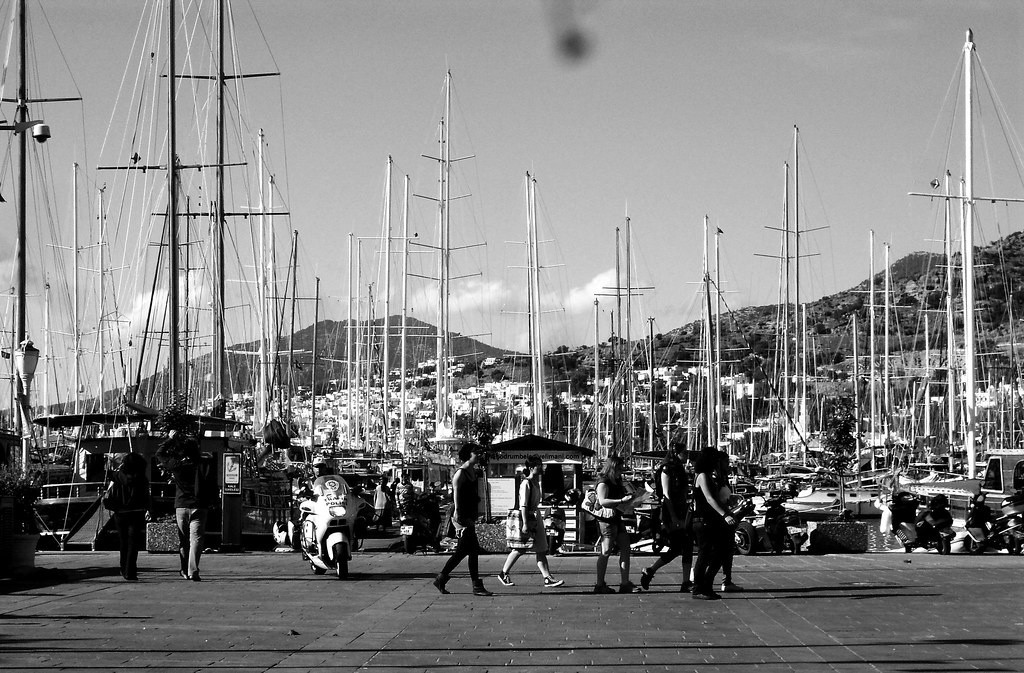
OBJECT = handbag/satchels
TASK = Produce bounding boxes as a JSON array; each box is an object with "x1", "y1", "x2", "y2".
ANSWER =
[
  {"x1": 101, "y1": 491, "x2": 113, "y2": 510},
  {"x1": 684, "y1": 503, "x2": 694, "y2": 534},
  {"x1": 442, "y1": 504, "x2": 458, "y2": 539},
  {"x1": 194, "y1": 459, "x2": 216, "y2": 499},
  {"x1": 506, "y1": 509, "x2": 535, "y2": 550},
  {"x1": 581, "y1": 482, "x2": 616, "y2": 521}
]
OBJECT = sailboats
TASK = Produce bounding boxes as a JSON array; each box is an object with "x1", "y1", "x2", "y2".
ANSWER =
[{"x1": 0, "y1": 0, "x2": 1024, "y2": 549}]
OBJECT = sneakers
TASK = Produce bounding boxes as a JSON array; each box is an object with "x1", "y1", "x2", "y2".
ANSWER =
[
  {"x1": 544, "y1": 574, "x2": 564, "y2": 587},
  {"x1": 498, "y1": 571, "x2": 515, "y2": 586}
]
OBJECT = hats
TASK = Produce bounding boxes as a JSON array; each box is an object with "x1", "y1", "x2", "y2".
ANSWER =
[
  {"x1": 459, "y1": 443, "x2": 477, "y2": 462},
  {"x1": 313, "y1": 457, "x2": 327, "y2": 466}
]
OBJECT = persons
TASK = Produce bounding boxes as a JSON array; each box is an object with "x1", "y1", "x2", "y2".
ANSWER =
[
  {"x1": 641, "y1": 442, "x2": 741, "y2": 600},
  {"x1": 594, "y1": 454, "x2": 641, "y2": 592},
  {"x1": 106, "y1": 452, "x2": 150, "y2": 580},
  {"x1": 296, "y1": 457, "x2": 414, "y2": 535},
  {"x1": 498, "y1": 458, "x2": 565, "y2": 588},
  {"x1": 154, "y1": 429, "x2": 213, "y2": 582},
  {"x1": 433, "y1": 443, "x2": 492, "y2": 596}
]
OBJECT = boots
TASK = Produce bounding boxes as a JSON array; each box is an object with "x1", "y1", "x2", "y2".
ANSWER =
[
  {"x1": 472, "y1": 579, "x2": 493, "y2": 596},
  {"x1": 433, "y1": 572, "x2": 451, "y2": 594}
]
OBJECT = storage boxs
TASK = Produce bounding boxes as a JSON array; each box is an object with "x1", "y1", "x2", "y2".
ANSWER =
[{"x1": 1001, "y1": 496, "x2": 1024, "y2": 516}]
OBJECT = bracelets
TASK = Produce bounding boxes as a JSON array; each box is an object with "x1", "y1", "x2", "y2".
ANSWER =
[
  {"x1": 621, "y1": 498, "x2": 623, "y2": 504},
  {"x1": 723, "y1": 514, "x2": 727, "y2": 519}
]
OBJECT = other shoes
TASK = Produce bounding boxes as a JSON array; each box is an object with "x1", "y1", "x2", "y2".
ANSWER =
[
  {"x1": 692, "y1": 588, "x2": 721, "y2": 600},
  {"x1": 640, "y1": 567, "x2": 656, "y2": 590},
  {"x1": 594, "y1": 582, "x2": 616, "y2": 594},
  {"x1": 179, "y1": 569, "x2": 202, "y2": 582},
  {"x1": 119, "y1": 567, "x2": 139, "y2": 583},
  {"x1": 722, "y1": 583, "x2": 744, "y2": 592},
  {"x1": 619, "y1": 581, "x2": 642, "y2": 594},
  {"x1": 681, "y1": 581, "x2": 694, "y2": 593}
]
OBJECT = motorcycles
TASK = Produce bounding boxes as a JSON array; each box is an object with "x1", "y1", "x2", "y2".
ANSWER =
[{"x1": 288, "y1": 474, "x2": 366, "y2": 580}]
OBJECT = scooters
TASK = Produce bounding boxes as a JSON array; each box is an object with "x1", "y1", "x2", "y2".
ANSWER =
[
  {"x1": 399, "y1": 479, "x2": 443, "y2": 554},
  {"x1": 595, "y1": 482, "x2": 761, "y2": 555},
  {"x1": 888, "y1": 489, "x2": 956, "y2": 556},
  {"x1": 539, "y1": 489, "x2": 567, "y2": 557},
  {"x1": 744, "y1": 483, "x2": 809, "y2": 557},
  {"x1": 962, "y1": 483, "x2": 1024, "y2": 556}
]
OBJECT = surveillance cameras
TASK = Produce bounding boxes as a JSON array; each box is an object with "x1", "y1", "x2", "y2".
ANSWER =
[{"x1": 32, "y1": 124, "x2": 51, "y2": 143}]
